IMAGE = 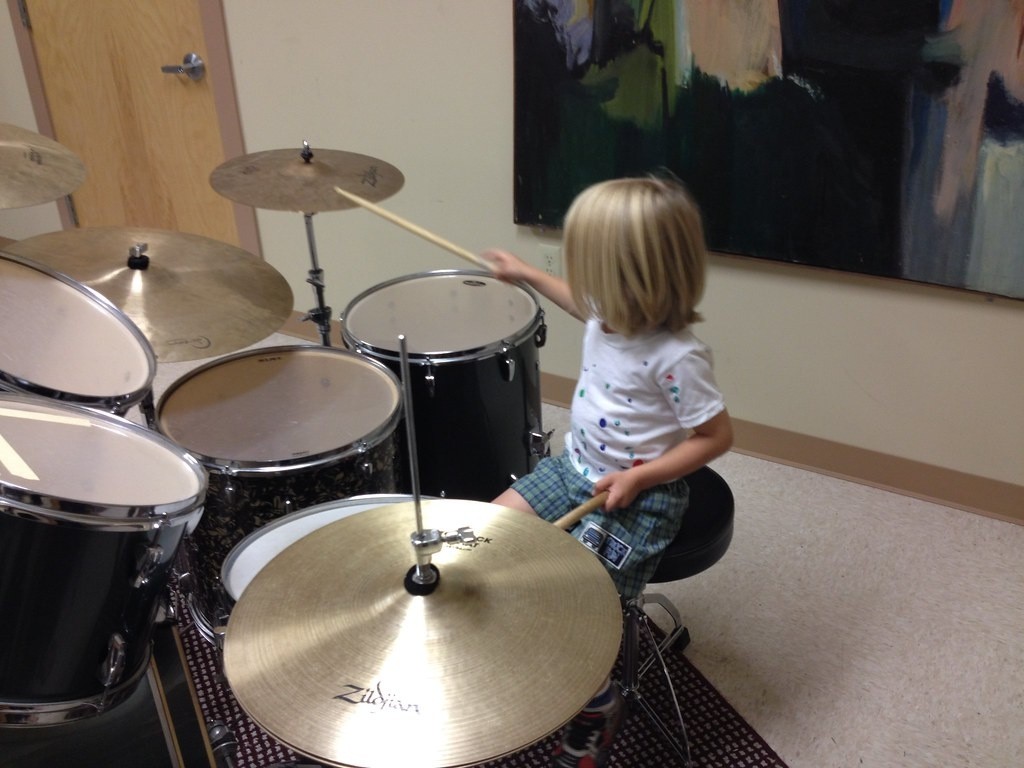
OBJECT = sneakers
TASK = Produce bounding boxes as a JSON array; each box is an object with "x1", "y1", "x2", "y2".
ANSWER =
[{"x1": 552, "y1": 686, "x2": 626, "y2": 768}]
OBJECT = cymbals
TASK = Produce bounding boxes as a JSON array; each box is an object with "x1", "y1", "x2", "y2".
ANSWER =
[
  {"x1": 0, "y1": 125, "x2": 88, "y2": 211},
  {"x1": 208, "y1": 148, "x2": 406, "y2": 213},
  {"x1": 0, "y1": 227, "x2": 294, "y2": 364},
  {"x1": 222, "y1": 499, "x2": 624, "y2": 767}
]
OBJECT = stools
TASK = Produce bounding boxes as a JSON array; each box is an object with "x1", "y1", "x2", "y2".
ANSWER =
[{"x1": 618, "y1": 462, "x2": 735, "y2": 768}]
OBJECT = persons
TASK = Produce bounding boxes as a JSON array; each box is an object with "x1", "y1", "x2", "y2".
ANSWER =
[{"x1": 480, "y1": 180, "x2": 734, "y2": 768}]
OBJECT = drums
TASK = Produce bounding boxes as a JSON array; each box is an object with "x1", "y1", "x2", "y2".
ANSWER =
[
  {"x1": 340, "y1": 270, "x2": 550, "y2": 504},
  {"x1": 0, "y1": 250, "x2": 158, "y2": 418},
  {"x1": 156, "y1": 346, "x2": 402, "y2": 652},
  {"x1": 0, "y1": 397, "x2": 209, "y2": 730},
  {"x1": 221, "y1": 494, "x2": 440, "y2": 616}
]
